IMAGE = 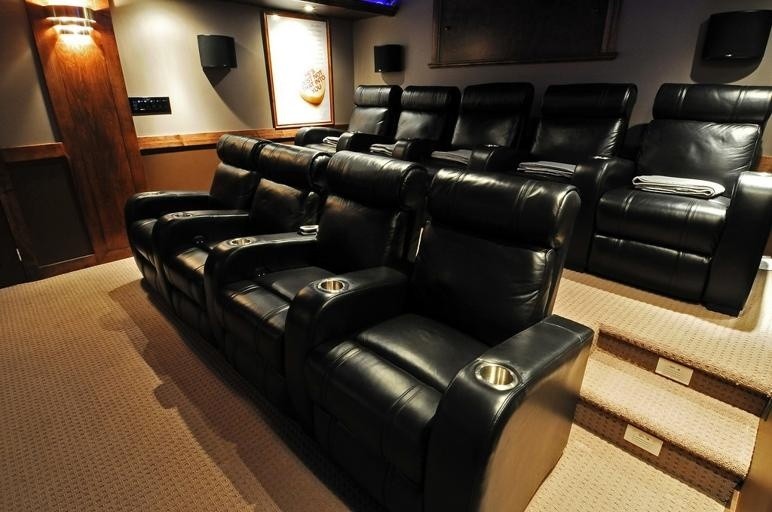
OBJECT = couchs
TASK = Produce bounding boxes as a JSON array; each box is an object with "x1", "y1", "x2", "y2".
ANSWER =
[
  {"x1": 294, "y1": 82, "x2": 772, "y2": 317},
  {"x1": 122, "y1": 133, "x2": 595, "y2": 511}
]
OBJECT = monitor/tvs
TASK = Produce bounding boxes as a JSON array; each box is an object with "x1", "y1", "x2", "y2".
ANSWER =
[{"x1": 304, "y1": 0, "x2": 403, "y2": 17}]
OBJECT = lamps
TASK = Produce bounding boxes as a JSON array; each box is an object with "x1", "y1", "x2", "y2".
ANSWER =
[{"x1": 26, "y1": 0, "x2": 117, "y2": 50}]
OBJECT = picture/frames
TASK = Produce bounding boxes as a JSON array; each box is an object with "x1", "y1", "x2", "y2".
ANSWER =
[{"x1": 261, "y1": 9, "x2": 335, "y2": 130}]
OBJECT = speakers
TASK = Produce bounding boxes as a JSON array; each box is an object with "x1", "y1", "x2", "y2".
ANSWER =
[
  {"x1": 699, "y1": 10, "x2": 771, "y2": 66},
  {"x1": 197, "y1": 34, "x2": 238, "y2": 70},
  {"x1": 374, "y1": 44, "x2": 404, "y2": 73}
]
